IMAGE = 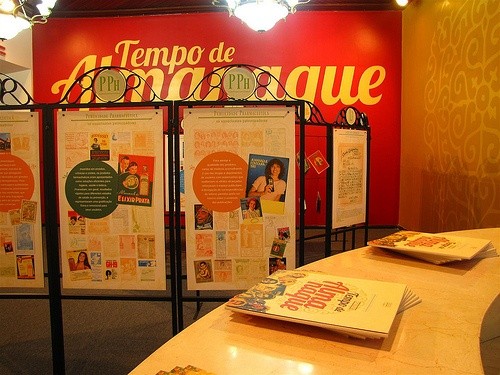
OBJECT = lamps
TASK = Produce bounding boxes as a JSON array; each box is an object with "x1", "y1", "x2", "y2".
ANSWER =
[
  {"x1": 212, "y1": 0, "x2": 311, "y2": 33},
  {"x1": 0, "y1": 0, "x2": 57, "y2": 42}
]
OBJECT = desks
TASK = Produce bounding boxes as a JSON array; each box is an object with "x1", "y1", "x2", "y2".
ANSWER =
[{"x1": 128, "y1": 228, "x2": 500, "y2": 375}]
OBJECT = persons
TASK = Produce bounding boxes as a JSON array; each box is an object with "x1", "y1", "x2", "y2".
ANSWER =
[
  {"x1": 74, "y1": 252, "x2": 91, "y2": 271},
  {"x1": 242, "y1": 197, "x2": 260, "y2": 219},
  {"x1": 156, "y1": 365, "x2": 215, "y2": 375},
  {"x1": 246, "y1": 159, "x2": 287, "y2": 203}
]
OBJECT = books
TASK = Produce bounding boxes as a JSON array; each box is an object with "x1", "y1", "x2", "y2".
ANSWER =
[
  {"x1": 366, "y1": 228, "x2": 500, "y2": 266},
  {"x1": 224, "y1": 269, "x2": 422, "y2": 341}
]
